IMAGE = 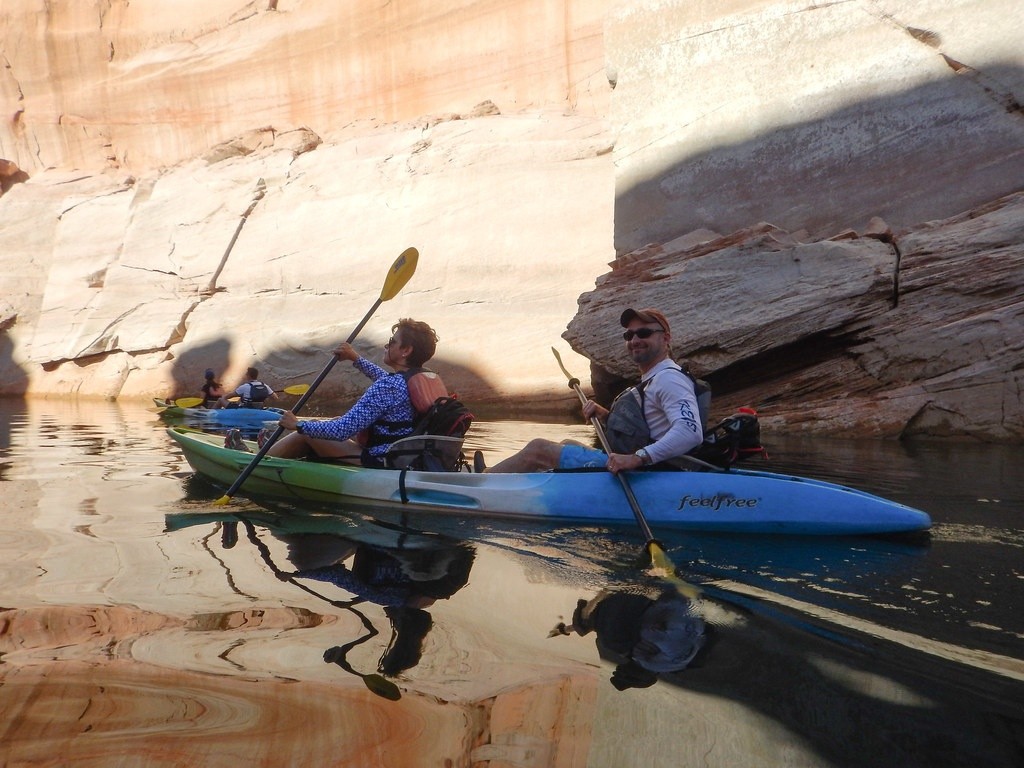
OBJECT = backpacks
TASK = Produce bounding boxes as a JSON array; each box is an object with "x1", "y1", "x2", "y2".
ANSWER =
[
  {"x1": 408, "y1": 393, "x2": 473, "y2": 438},
  {"x1": 692, "y1": 409, "x2": 767, "y2": 468}
]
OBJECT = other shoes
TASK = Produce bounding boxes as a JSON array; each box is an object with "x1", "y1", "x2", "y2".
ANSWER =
[{"x1": 474, "y1": 450, "x2": 486, "y2": 473}]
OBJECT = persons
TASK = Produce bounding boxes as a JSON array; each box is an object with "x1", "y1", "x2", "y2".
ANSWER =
[
  {"x1": 212, "y1": 368, "x2": 278, "y2": 410},
  {"x1": 225, "y1": 318, "x2": 437, "y2": 469},
  {"x1": 483, "y1": 309, "x2": 705, "y2": 473},
  {"x1": 201, "y1": 368, "x2": 222, "y2": 409}
]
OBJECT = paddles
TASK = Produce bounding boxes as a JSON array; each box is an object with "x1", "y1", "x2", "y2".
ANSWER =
[
  {"x1": 551, "y1": 347, "x2": 672, "y2": 568},
  {"x1": 146, "y1": 396, "x2": 238, "y2": 414},
  {"x1": 175, "y1": 384, "x2": 310, "y2": 409},
  {"x1": 212, "y1": 248, "x2": 420, "y2": 505}
]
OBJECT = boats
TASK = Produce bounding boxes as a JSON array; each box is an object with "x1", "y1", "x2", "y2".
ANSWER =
[
  {"x1": 152, "y1": 395, "x2": 292, "y2": 429},
  {"x1": 166, "y1": 421, "x2": 935, "y2": 538}
]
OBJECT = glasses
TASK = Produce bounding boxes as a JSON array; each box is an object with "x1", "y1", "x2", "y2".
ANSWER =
[
  {"x1": 389, "y1": 338, "x2": 396, "y2": 348},
  {"x1": 245, "y1": 373, "x2": 248, "y2": 375},
  {"x1": 623, "y1": 327, "x2": 665, "y2": 341}
]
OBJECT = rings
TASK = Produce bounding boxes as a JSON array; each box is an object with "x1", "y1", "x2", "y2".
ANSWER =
[{"x1": 609, "y1": 466, "x2": 613, "y2": 469}]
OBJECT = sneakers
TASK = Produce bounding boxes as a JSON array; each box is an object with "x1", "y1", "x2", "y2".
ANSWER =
[
  {"x1": 224, "y1": 428, "x2": 251, "y2": 452},
  {"x1": 257, "y1": 427, "x2": 273, "y2": 449}
]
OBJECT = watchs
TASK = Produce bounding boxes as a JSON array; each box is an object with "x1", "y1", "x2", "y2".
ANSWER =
[{"x1": 635, "y1": 449, "x2": 650, "y2": 466}]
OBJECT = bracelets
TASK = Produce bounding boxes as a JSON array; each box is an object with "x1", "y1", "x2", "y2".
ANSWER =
[{"x1": 297, "y1": 421, "x2": 305, "y2": 434}]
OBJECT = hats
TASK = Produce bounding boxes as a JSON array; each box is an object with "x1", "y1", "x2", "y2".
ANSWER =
[{"x1": 620, "y1": 308, "x2": 670, "y2": 334}]
